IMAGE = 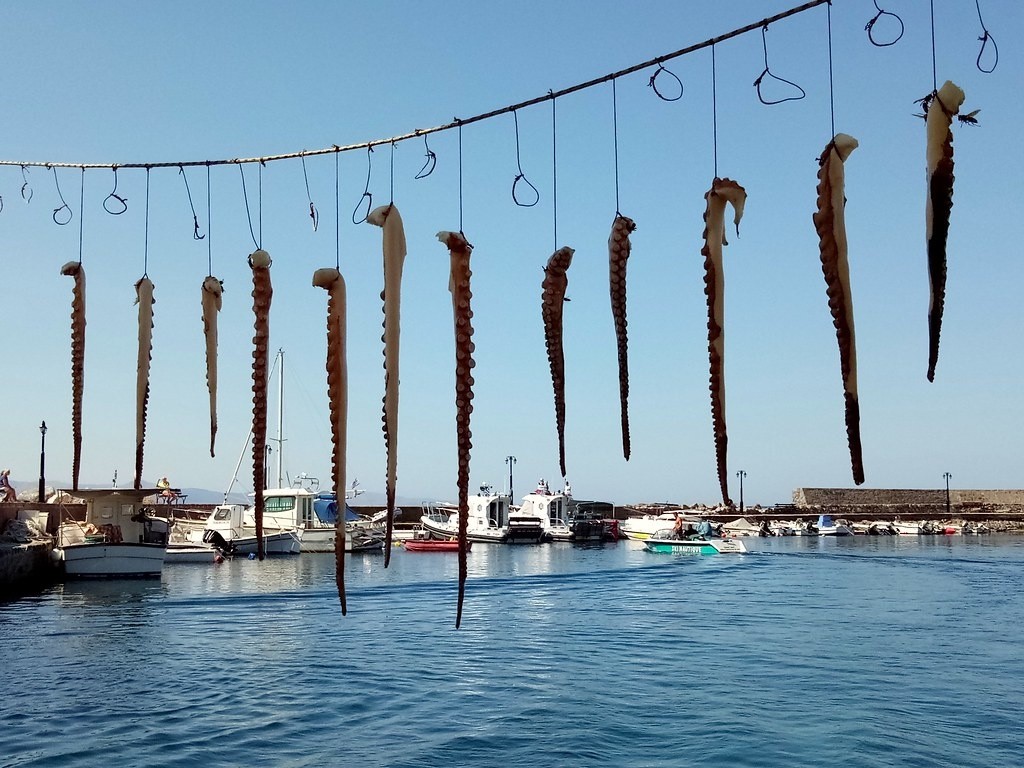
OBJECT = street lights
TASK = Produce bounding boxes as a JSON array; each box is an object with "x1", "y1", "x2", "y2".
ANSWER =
[
  {"x1": 505, "y1": 455, "x2": 517, "y2": 505},
  {"x1": 39, "y1": 420, "x2": 48, "y2": 503},
  {"x1": 736, "y1": 469, "x2": 746, "y2": 515},
  {"x1": 942, "y1": 471, "x2": 952, "y2": 513},
  {"x1": 264, "y1": 444, "x2": 272, "y2": 490}
]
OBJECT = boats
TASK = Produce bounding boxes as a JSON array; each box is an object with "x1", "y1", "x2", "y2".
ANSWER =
[
  {"x1": 721, "y1": 516, "x2": 992, "y2": 538},
  {"x1": 165, "y1": 344, "x2": 432, "y2": 565},
  {"x1": 619, "y1": 511, "x2": 714, "y2": 539},
  {"x1": 513, "y1": 477, "x2": 628, "y2": 542},
  {"x1": 419, "y1": 481, "x2": 553, "y2": 545},
  {"x1": 641, "y1": 527, "x2": 749, "y2": 555},
  {"x1": 53, "y1": 487, "x2": 181, "y2": 581},
  {"x1": 403, "y1": 533, "x2": 474, "y2": 553}
]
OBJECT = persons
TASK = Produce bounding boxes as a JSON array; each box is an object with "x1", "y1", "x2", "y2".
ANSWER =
[
  {"x1": 0, "y1": 469, "x2": 19, "y2": 502},
  {"x1": 159, "y1": 477, "x2": 177, "y2": 504},
  {"x1": 673, "y1": 512, "x2": 682, "y2": 536},
  {"x1": 684, "y1": 524, "x2": 698, "y2": 536}
]
